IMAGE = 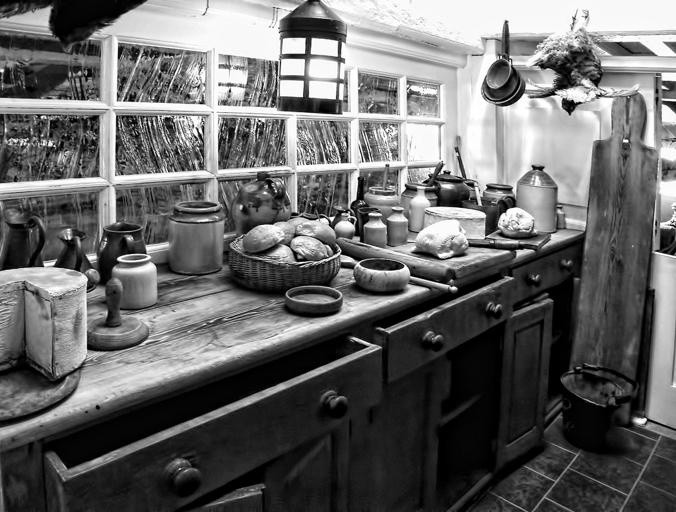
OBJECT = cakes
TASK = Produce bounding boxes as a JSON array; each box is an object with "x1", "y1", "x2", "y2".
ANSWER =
[{"x1": 0, "y1": 268, "x2": 88, "y2": 381}]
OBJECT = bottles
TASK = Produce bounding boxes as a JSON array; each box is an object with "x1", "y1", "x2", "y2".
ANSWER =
[
  {"x1": 557, "y1": 205, "x2": 565, "y2": 229},
  {"x1": 111, "y1": 254, "x2": 158, "y2": 310},
  {"x1": 364, "y1": 213, "x2": 386, "y2": 247},
  {"x1": 387, "y1": 206, "x2": 408, "y2": 246},
  {"x1": 409, "y1": 186, "x2": 431, "y2": 233},
  {"x1": 350, "y1": 176, "x2": 369, "y2": 236},
  {"x1": 515, "y1": 164, "x2": 558, "y2": 234},
  {"x1": 167, "y1": 200, "x2": 226, "y2": 274},
  {"x1": 483, "y1": 183, "x2": 515, "y2": 199}
]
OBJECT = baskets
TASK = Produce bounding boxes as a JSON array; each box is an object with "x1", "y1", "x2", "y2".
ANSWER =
[{"x1": 228, "y1": 235, "x2": 342, "y2": 292}]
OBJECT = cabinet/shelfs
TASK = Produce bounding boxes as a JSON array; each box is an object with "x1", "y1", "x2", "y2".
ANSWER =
[
  {"x1": 512, "y1": 238, "x2": 585, "y2": 470},
  {"x1": 651, "y1": 74, "x2": 675, "y2": 248},
  {"x1": 385, "y1": 270, "x2": 511, "y2": 512},
  {"x1": 0, "y1": 316, "x2": 384, "y2": 512}
]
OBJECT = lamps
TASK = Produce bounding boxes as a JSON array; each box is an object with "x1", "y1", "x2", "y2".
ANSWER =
[{"x1": 269, "y1": 3, "x2": 346, "y2": 112}]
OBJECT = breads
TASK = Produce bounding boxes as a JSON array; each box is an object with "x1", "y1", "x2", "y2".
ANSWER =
[{"x1": 242, "y1": 216, "x2": 337, "y2": 263}]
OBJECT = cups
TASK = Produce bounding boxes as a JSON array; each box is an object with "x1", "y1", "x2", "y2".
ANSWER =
[{"x1": 358, "y1": 208, "x2": 380, "y2": 242}]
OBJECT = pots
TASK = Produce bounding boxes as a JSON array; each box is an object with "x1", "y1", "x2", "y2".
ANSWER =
[{"x1": 481, "y1": 19, "x2": 526, "y2": 107}]
